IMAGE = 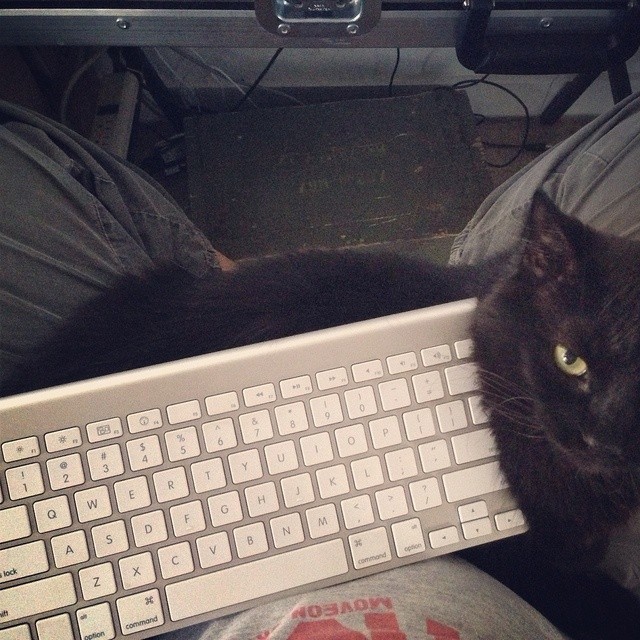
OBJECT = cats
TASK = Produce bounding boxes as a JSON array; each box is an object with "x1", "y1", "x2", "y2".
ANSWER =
[{"x1": 0, "y1": 187, "x2": 640, "y2": 639}]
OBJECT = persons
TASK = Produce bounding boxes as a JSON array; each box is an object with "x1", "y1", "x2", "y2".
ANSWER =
[{"x1": 1, "y1": 93, "x2": 640, "y2": 640}]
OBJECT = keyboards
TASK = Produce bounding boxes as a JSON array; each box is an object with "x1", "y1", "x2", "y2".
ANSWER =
[{"x1": 0, "y1": 296, "x2": 535, "y2": 639}]
